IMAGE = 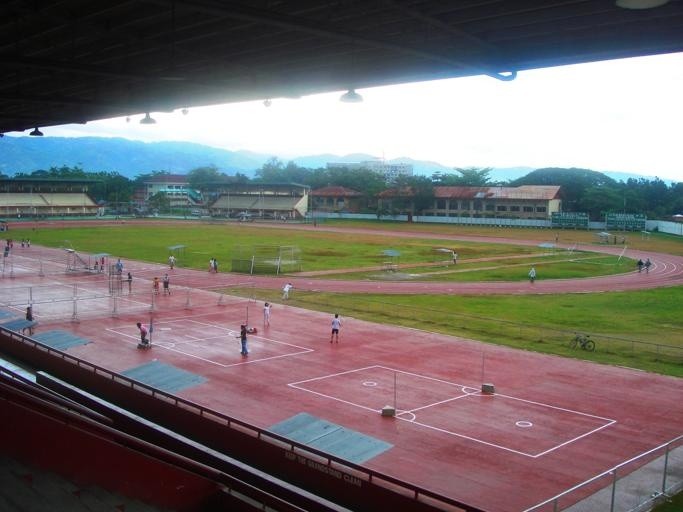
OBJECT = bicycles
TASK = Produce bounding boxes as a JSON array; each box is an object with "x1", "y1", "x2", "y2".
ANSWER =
[{"x1": 569, "y1": 333, "x2": 596, "y2": 353}]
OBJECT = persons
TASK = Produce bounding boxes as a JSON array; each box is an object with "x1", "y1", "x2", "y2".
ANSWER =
[
  {"x1": 637, "y1": 259, "x2": 644, "y2": 272},
  {"x1": 94, "y1": 258, "x2": 105, "y2": 272},
  {"x1": 7, "y1": 238, "x2": 31, "y2": 249},
  {"x1": 331, "y1": 313, "x2": 341, "y2": 342},
  {"x1": 137, "y1": 323, "x2": 149, "y2": 344},
  {"x1": 621, "y1": 236, "x2": 625, "y2": 243},
  {"x1": 281, "y1": 282, "x2": 294, "y2": 300},
  {"x1": 27, "y1": 307, "x2": 36, "y2": 330},
  {"x1": 263, "y1": 303, "x2": 271, "y2": 328},
  {"x1": 209, "y1": 257, "x2": 217, "y2": 273},
  {"x1": 273, "y1": 213, "x2": 286, "y2": 221},
  {"x1": 153, "y1": 273, "x2": 171, "y2": 297},
  {"x1": 169, "y1": 254, "x2": 175, "y2": 269},
  {"x1": 115, "y1": 258, "x2": 123, "y2": 274},
  {"x1": 128, "y1": 272, "x2": 132, "y2": 290},
  {"x1": 644, "y1": 257, "x2": 651, "y2": 273},
  {"x1": 529, "y1": 268, "x2": 535, "y2": 281},
  {"x1": 453, "y1": 250, "x2": 458, "y2": 264},
  {"x1": 236, "y1": 324, "x2": 248, "y2": 355}
]
